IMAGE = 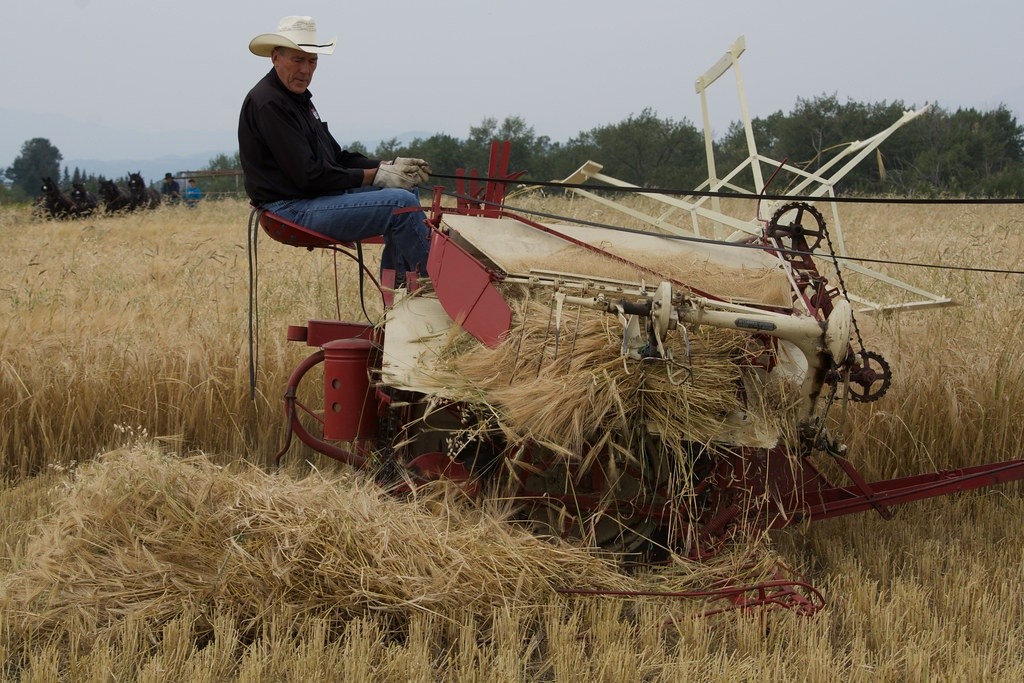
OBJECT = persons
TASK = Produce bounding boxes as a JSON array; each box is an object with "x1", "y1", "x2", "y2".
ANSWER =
[
  {"x1": 161, "y1": 172, "x2": 180, "y2": 205},
  {"x1": 235, "y1": 13, "x2": 435, "y2": 288},
  {"x1": 182, "y1": 179, "x2": 203, "y2": 206}
]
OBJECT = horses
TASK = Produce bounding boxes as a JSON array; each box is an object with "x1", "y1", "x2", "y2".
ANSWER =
[{"x1": 27, "y1": 170, "x2": 161, "y2": 221}]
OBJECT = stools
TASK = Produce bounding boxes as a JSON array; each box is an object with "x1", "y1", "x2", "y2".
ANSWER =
[{"x1": 249, "y1": 201, "x2": 385, "y2": 248}]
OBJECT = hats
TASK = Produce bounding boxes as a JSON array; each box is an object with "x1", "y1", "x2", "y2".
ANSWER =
[
  {"x1": 250, "y1": 12, "x2": 335, "y2": 60},
  {"x1": 163, "y1": 173, "x2": 175, "y2": 181}
]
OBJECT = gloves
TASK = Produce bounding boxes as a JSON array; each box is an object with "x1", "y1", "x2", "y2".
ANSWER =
[{"x1": 376, "y1": 157, "x2": 430, "y2": 191}]
